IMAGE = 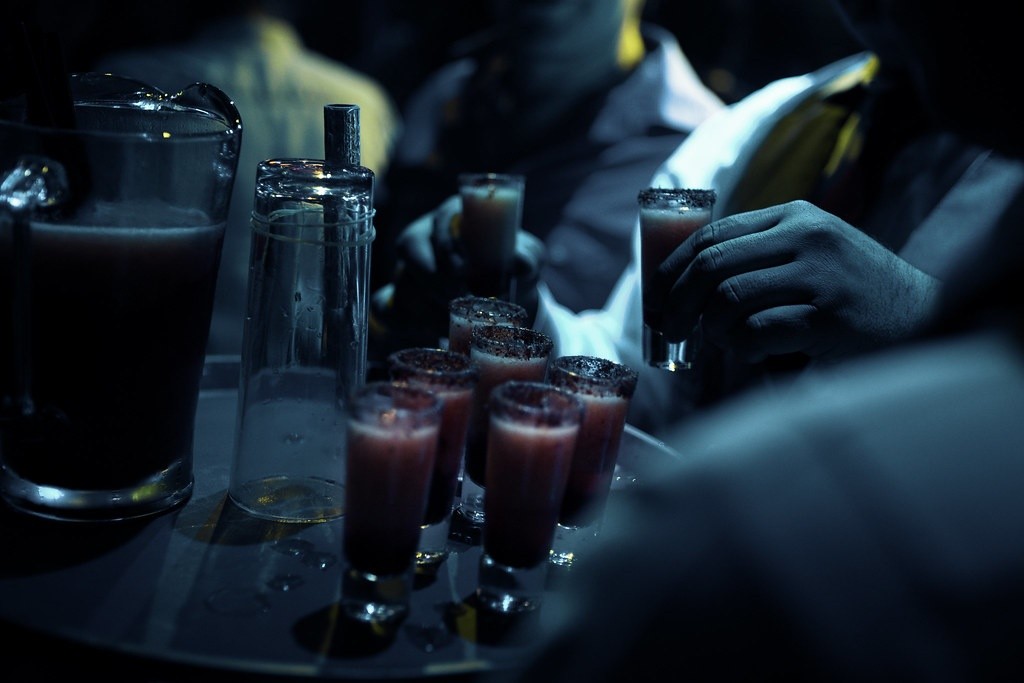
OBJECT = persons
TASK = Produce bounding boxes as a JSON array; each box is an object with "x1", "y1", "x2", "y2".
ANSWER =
[{"x1": 124, "y1": 0, "x2": 1024, "y2": 683}]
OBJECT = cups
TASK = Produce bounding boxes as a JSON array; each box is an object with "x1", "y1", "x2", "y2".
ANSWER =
[
  {"x1": 550, "y1": 356, "x2": 637, "y2": 567},
  {"x1": 340, "y1": 382, "x2": 440, "y2": 621},
  {"x1": 460, "y1": 326, "x2": 552, "y2": 523},
  {"x1": 1, "y1": 98, "x2": 240, "y2": 521},
  {"x1": 639, "y1": 188, "x2": 716, "y2": 371},
  {"x1": 232, "y1": 158, "x2": 373, "y2": 524},
  {"x1": 450, "y1": 298, "x2": 525, "y2": 480},
  {"x1": 478, "y1": 383, "x2": 583, "y2": 613},
  {"x1": 458, "y1": 172, "x2": 524, "y2": 278},
  {"x1": 391, "y1": 347, "x2": 479, "y2": 563}
]
